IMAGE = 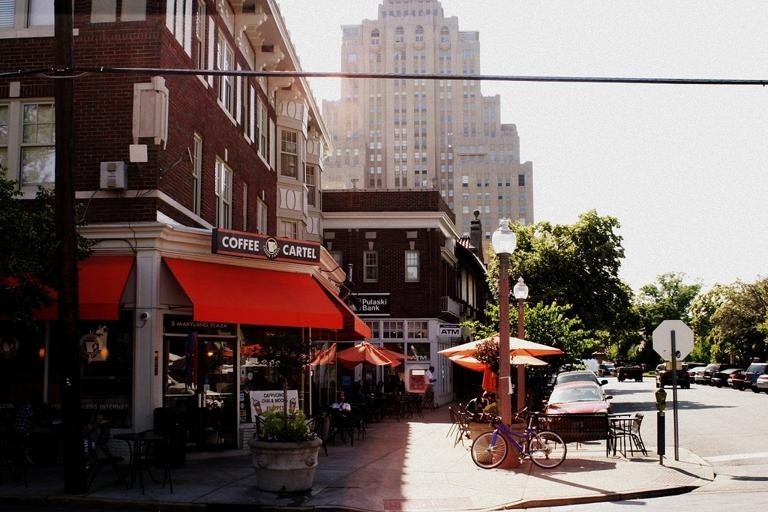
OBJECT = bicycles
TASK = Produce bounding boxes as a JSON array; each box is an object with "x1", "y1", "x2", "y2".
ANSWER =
[{"x1": 471, "y1": 408, "x2": 570, "y2": 473}]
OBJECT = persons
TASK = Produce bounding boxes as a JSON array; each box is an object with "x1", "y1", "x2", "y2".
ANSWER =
[
  {"x1": 465, "y1": 391, "x2": 489, "y2": 417},
  {"x1": 424, "y1": 367, "x2": 437, "y2": 409},
  {"x1": 332, "y1": 391, "x2": 351, "y2": 444},
  {"x1": 482, "y1": 390, "x2": 495, "y2": 398}
]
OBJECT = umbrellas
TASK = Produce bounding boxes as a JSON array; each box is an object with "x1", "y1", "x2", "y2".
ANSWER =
[
  {"x1": 437, "y1": 333, "x2": 564, "y2": 356},
  {"x1": 449, "y1": 355, "x2": 548, "y2": 367},
  {"x1": 338, "y1": 341, "x2": 406, "y2": 394}
]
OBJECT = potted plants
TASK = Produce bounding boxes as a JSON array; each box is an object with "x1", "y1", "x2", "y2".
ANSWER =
[{"x1": 245, "y1": 404, "x2": 323, "y2": 495}]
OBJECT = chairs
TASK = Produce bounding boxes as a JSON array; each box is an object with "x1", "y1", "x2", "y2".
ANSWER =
[
  {"x1": 380, "y1": 389, "x2": 435, "y2": 423},
  {"x1": 314, "y1": 392, "x2": 380, "y2": 449},
  {"x1": 1, "y1": 412, "x2": 174, "y2": 496},
  {"x1": 444, "y1": 398, "x2": 497, "y2": 449}
]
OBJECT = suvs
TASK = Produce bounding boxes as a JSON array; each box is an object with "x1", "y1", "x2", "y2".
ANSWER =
[
  {"x1": 554, "y1": 369, "x2": 608, "y2": 390},
  {"x1": 167, "y1": 374, "x2": 188, "y2": 395}
]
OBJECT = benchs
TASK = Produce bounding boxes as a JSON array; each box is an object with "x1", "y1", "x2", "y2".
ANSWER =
[{"x1": 606, "y1": 410, "x2": 647, "y2": 459}]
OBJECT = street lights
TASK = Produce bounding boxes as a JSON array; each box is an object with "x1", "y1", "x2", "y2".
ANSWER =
[
  {"x1": 491, "y1": 213, "x2": 517, "y2": 428},
  {"x1": 510, "y1": 276, "x2": 529, "y2": 419}
]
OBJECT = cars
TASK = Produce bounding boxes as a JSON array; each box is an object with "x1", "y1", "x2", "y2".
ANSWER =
[
  {"x1": 545, "y1": 382, "x2": 613, "y2": 437},
  {"x1": 597, "y1": 360, "x2": 645, "y2": 383},
  {"x1": 654, "y1": 360, "x2": 767, "y2": 396}
]
